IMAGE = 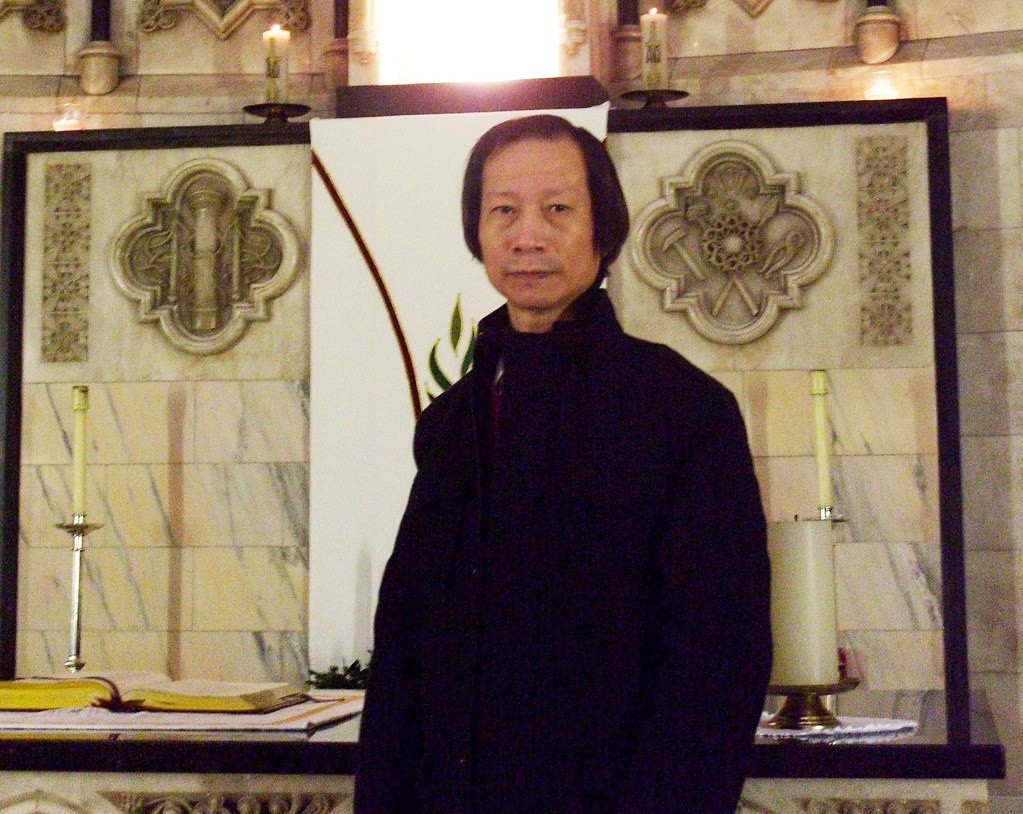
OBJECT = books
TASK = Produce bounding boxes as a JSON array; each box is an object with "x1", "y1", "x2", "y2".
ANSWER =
[{"x1": 0, "y1": 677, "x2": 305, "y2": 715}]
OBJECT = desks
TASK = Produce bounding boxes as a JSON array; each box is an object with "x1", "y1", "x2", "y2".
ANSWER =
[{"x1": 1, "y1": 690, "x2": 1008, "y2": 813}]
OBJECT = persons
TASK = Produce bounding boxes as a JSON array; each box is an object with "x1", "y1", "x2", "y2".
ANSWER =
[{"x1": 351, "y1": 114, "x2": 774, "y2": 814}]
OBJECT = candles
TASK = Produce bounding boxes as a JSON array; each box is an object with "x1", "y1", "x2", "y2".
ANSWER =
[
  {"x1": 639, "y1": 7, "x2": 669, "y2": 89},
  {"x1": 264, "y1": 22, "x2": 292, "y2": 103},
  {"x1": 71, "y1": 386, "x2": 89, "y2": 513},
  {"x1": 809, "y1": 369, "x2": 833, "y2": 505}
]
